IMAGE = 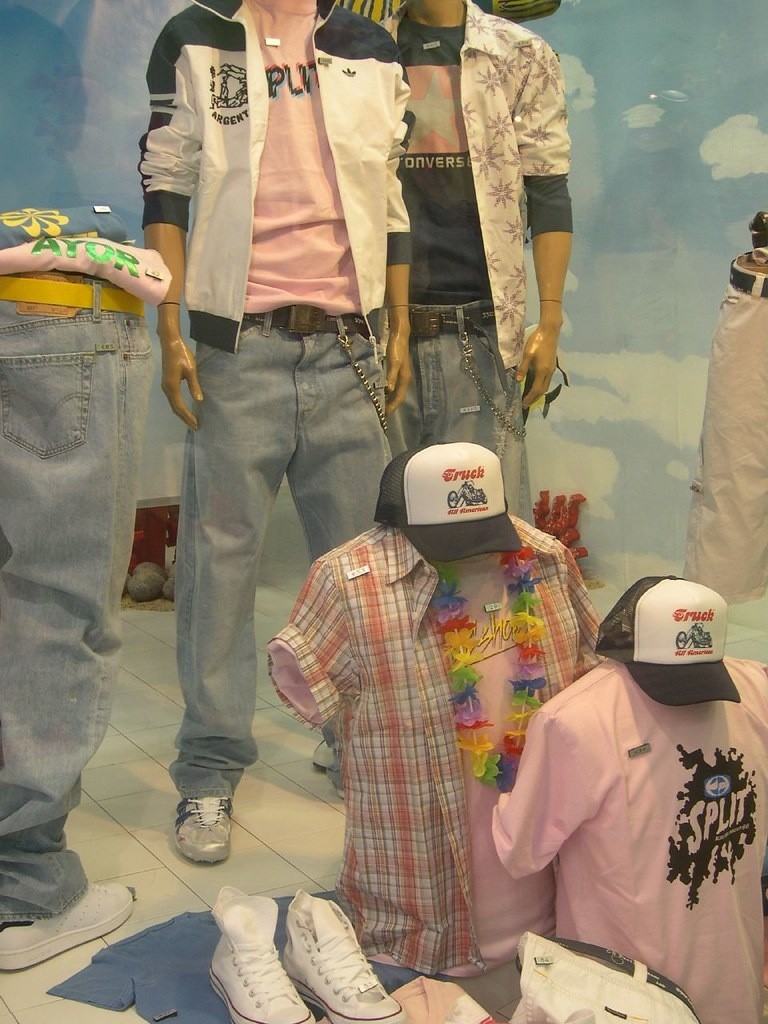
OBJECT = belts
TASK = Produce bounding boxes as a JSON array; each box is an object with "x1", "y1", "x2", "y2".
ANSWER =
[
  {"x1": 407, "y1": 304, "x2": 513, "y2": 396},
  {"x1": 730, "y1": 250, "x2": 768, "y2": 297},
  {"x1": 244, "y1": 303, "x2": 370, "y2": 338},
  {"x1": 516, "y1": 930, "x2": 700, "y2": 1023},
  {"x1": 0, "y1": 274, "x2": 147, "y2": 318}
]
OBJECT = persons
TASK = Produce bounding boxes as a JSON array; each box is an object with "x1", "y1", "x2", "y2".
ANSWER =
[
  {"x1": 683, "y1": 248, "x2": 768, "y2": 606},
  {"x1": 266, "y1": 444, "x2": 608, "y2": 979},
  {"x1": 0, "y1": 268, "x2": 151, "y2": 970},
  {"x1": 311, "y1": 0, "x2": 575, "y2": 771},
  {"x1": 493, "y1": 576, "x2": 768, "y2": 1024},
  {"x1": 137, "y1": 0, "x2": 411, "y2": 863}
]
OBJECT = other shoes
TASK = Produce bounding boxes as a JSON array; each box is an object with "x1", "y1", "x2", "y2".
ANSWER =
[{"x1": 313, "y1": 735, "x2": 335, "y2": 768}]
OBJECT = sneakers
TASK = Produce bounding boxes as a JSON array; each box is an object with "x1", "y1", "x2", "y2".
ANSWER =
[
  {"x1": 210, "y1": 886, "x2": 315, "y2": 1024},
  {"x1": 282, "y1": 889, "x2": 405, "y2": 1024},
  {"x1": 0, "y1": 882, "x2": 134, "y2": 972},
  {"x1": 176, "y1": 793, "x2": 233, "y2": 862}
]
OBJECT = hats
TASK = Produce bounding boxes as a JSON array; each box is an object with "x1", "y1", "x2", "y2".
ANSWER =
[
  {"x1": 593, "y1": 574, "x2": 741, "y2": 705},
  {"x1": 373, "y1": 442, "x2": 521, "y2": 562}
]
